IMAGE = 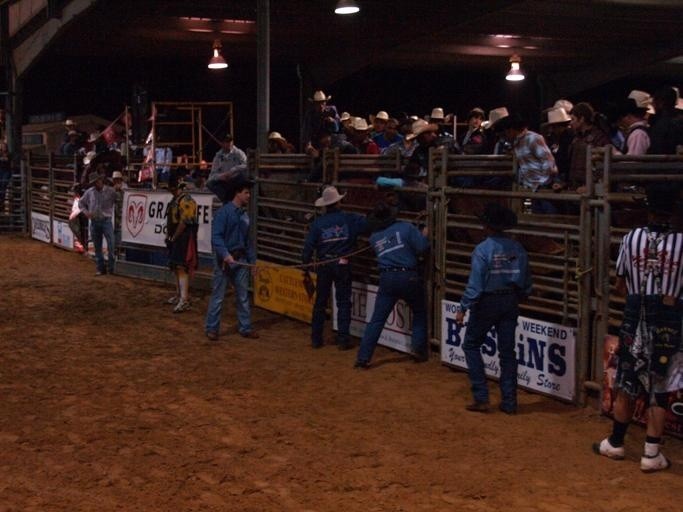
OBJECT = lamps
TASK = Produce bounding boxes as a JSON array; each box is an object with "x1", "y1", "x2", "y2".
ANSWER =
[
  {"x1": 505, "y1": 54, "x2": 525, "y2": 83},
  {"x1": 207, "y1": 37, "x2": 229, "y2": 70},
  {"x1": 334, "y1": 0, "x2": 361, "y2": 15}
]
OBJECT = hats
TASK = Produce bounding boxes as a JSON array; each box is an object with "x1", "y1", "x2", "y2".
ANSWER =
[
  {"x1": 268, "y1": 132, "x2": 285, "y2": 141},
  {"x1": 538, "y1": 81, "x2": 682, "y2": 126},
  {"x1": 63, "y1": 119, "x2": 125, "y2": 188},
  {"x1": 310, "y1": 92, "x2": 511, "y2": 141},
  {"x1": 215, "y1": 130, "x2": 236, "y2": 142},
  {"x1": 475, "y1": 203, "x2": 517, "y2": 229},
  {"x1": 167, "y1": 180, "x2": 186, "y2": 192},
  {"x1": 314, "y1": 186, "x2": 345, "y2": 206}
]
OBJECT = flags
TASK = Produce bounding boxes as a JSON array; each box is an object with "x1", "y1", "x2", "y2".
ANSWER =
[
  {"x1": 143, "y1": 146, "x2": 153, "y2": 162},
  {"x1": 137, "y1": 167, "x2": 153, "y2": 183},
  {"x1": 122, "y1": 111, "x2": 131, "y2": 127},
  {"x1": 102, "y1": 128, "x2": 117, "y2": 144},
  {"x1": 143, "y1": 128, "x2": 153, "y2": 144}
]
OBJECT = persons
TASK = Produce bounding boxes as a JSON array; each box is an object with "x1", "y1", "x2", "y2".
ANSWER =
[
  {"x1": 298, "y1": 185, "x2": 367, "y2": 350},
  {"x1": 268, "y1": 90, "x2": 682, "y2": 261},
  {"x1": 205, "y1": 178, "x2": 257, "y2": 339},
  {"x1": 209, "y1": 135, "x2": 247, "y2": 181},
  {"x1": 192, "y1": 160, "x2": 210, "y2": 178},
  {"x1": 593, "y1": 184, "x2": 681, "y2": 471},
  {"x1": 455, "y1": 205, "x2": 531, "y2": 416},
  {"x1": 109, "y1": 171, "x2": 131, "y2": 193},
  {"x1": 162, "y1": 175, "x2": 200, "y2": 315},
  {"x1": 0, "y1": 143, "x2": 11, "y2": 208},
  {"x1": 352, "y1": 202, "x2": 430, "y2": 369},
  {"x1": 120, "y1": 131, "x2": 135, "y2": 165},
  {"x1": 63, "y1": 130, "x2": 82, "y2": 168},
  {"x1": 87, "y1": 133, "x2": 109, "y2": 155},
  {"x1": 79, "y1": 172, "x2": 122, "y2": 279},
  {"x1": 141, "y1": 133, "x2": 173, "y2": 181},
  {"x1": 58, "y1": 118, "x2": 80, "y2": 156}
]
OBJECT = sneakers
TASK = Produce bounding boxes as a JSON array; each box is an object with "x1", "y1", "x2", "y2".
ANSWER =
[
  {"x1": 167, "y1": 294, "x2": 179, "y2": 305},
  {"x1": 174, "y1": 297, "x2": 191, "y2": 313}
]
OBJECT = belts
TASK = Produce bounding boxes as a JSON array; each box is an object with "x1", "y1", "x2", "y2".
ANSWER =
[{"x1": 381, "y1": 267, "x2": 410, "y2": 272}]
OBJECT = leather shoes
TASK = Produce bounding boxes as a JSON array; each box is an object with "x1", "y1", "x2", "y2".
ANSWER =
[
  {"x1": 499, "y1": 402, "x2": 518, "y2": 415},
  {"x1": 207, "y1": 332, "x2": 217, "y2": 340},
  {"x1": 352, "y1": 362, "x2": 368, "y2": 369},
  {"x1": 464, "y1": 401, "x2": 490, "y2": 412},
  {"x1": 639, "y1": 452, "x2": 671, "y2": 473},
  {"x1": 239, "y1": 330, "x2": 258, "y2": 338},
  {"x1": 591, "y1": 438, "x2": 624, "y2": 460},
  {"x1": 415, "y1": 348, "x2": 427, "y2": 362}
]
